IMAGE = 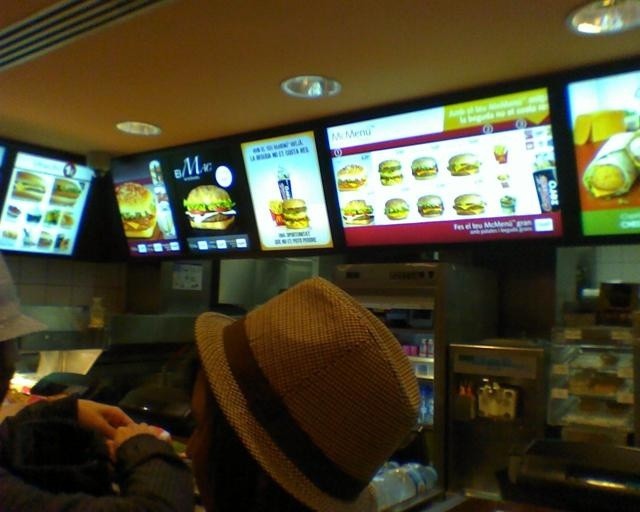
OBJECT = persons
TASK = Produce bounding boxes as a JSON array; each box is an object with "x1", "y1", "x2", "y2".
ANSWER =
[{"x1": 0, "y1": 250, "x2": 196, "y2": 512}]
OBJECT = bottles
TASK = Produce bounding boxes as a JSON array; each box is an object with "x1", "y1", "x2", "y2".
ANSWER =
[
  {"x1": 531, "y1": 152, "x2": 559, "y2": 213},
  {"x1": 90, "y1": 297, "x2": 106, "y2": 328},
  {"x1": 414, "y1": 379, "x2": 434, "y2": 425},
  {"x1": 360, "y1": 461, "x2": 440, "y2": 512},
  {"x1": 423, "y1": 338, "x2": 428, "y2": 356}
]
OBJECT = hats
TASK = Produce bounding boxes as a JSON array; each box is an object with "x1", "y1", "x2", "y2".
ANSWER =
[{"x1": 2, "y1": 255, "x2": 48, "y2": 345}]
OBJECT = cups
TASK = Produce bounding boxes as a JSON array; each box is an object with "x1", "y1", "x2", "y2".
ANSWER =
[
  {"x1": 278, "y1": 179, "x2": 292, "y2": 200},
  {"x1": 55, "y1": 233, "x2": 65, "y2": 248}
]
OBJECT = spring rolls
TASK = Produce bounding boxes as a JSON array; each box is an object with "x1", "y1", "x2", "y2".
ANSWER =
[{"x1": 582, "y1": 130, "x2": 640, "y2": 199}]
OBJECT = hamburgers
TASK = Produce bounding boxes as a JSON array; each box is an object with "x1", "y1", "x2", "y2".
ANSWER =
[
  {"x1": 114, "y1": 182, "x2": 157, "y2": 239},
  {"x1": 282, "y1": 198, "x2": 309, "y2": 229},
  {"x1": 51, "y1": 180, "x2": 82, "y2": 204},
  {"x1": 13, "y1": 172, "x2": 45, "y2": 201},
  {"x1": 183, "y1": 184, "x2": 236, "y2": 231},
  {"x1": 337, "y1": 153, "x2": 485, "y2": 226}
]
